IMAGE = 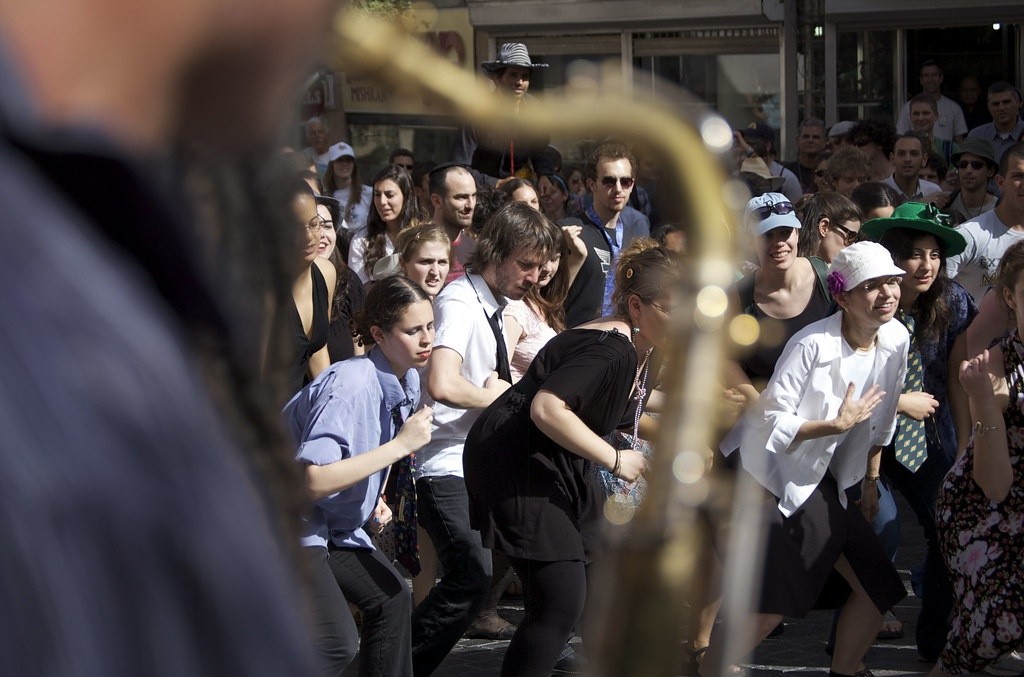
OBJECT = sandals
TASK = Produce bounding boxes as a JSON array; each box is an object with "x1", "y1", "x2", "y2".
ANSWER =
[{"x1": 874, "y1": 613, "x2": 905, "y2": 641}]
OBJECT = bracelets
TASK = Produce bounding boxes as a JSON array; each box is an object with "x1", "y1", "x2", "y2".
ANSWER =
[
  {"x1": 608, "y1": 447, "x2": 618, "y2": 475},
  {"x1": 613, "y1": 450, "x2": 622, "y2": 477},
  {"x1": 865, "y1": 473, "x2": 881, "y2": 481},
  {"x1": 973, "y1": 421, "x2": 1006, "y2": 438},
  {"x1": 747, "y1": 149, "x2": 755, "y2": 157}
]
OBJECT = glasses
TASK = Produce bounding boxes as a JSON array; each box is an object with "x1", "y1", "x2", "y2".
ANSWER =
[
  {"x1": 817, "y1": 217, "x2": 860, "y2": 246},
  {"x1": 814, "y1": 168, "x2": 827, "y2": 178},
  {"x1": 829, "y1": 137, "x2": 855, "y2": 148},
  {"x1": 567, "y1": 174, "x2": 586, "y2": 185},
  {"x1": 845, "y1": 276, "x2": 904, "y2": 297},
  {"x1": 399, "y1": 164, "x2": 415, "y2": 171},
  {"x1": 292, "y1": 220, "x2": 323, "y2": 234},
  {"x1": 595, "y1": 175, "x2": 635, "y2": 192},
  {"x1": 746, "y1": 202, "x2": 796, "y2": 227},
  {"x1": 855, "y1": 136, "x2": 876, "y2": 148},
  {"x1": 957, "y1": 159, "x2": 988, "y2": 170},
  {"x1": 627, "y1": 287, "x2": 679, "y2": 321}
]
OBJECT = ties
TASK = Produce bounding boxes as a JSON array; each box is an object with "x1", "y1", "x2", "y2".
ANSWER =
[
  {"x1": 894, "y1": 305, "x2": 928, "y2": 474},
  {"x1": 464, "y1": 271, "x2": 513, "y2": 388},
  {"x1": 586, "y1": 207, "x2": 624, "y2": 318},
  {"x1": 389, "y1": 399, "x2": 420, "y2": 577}
]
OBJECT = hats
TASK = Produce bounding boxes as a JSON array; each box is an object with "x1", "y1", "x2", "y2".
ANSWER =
[
  {"x1": 328, "y1": 141, "x2": 355, "y2": 165},
  {"x1": 741, "y1": 192, "x2": 804, "y2": 238},
  {"x1": 479, "y1": 42, "x2": 550, "y2": 80},
  {"x1": 827, "y1": 242, "x2": 906, "y2": 304},
  {"x1": 314, "y1": 192, "x2": 343, "y2": 232},
  {"x1": 860, "y1": 200, "x2": 968, "y2": 259},
  {"x1": 951, "y1": 137, "x2": 996, "y2": 169},
  {"x1": 828, "y1": 121, "x2": 860, "y2": 136}
]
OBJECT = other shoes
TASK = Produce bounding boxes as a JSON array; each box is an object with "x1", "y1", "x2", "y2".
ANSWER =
[
  {"x1": 464, "y1": 616, "x2": 518, "y2": 642},
  {"x1": 681, "y1": 642, "x2": 708, "y2": 677},
  {"x1": 826, "y1": 667, "x2": 877, "y2": 677},
  {"x1": 552, "y1": 647, "x2": 590, "y2": 676}
]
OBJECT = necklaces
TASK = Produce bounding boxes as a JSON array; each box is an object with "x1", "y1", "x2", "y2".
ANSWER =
[
  {"x1": 849, "y1": 337, "x2": 877, "y2": 350},
  {"x1": 960, "y1": 187, "x2": 988, "y2": 219},
  {"x1": 796, "y1": 161, "x2": 814, "y2": 194},
  {"x1": 632, "y1": 329, "x2": 650, "y2": 447}
]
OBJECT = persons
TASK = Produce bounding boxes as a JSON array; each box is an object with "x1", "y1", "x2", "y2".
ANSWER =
[
  {"x1": 275, "y1": 55, "x2": 1024, "y2": 677},
  {"x1": 0, "y1": 0, "x2": 366, "y2": 677},
  {"x1": 446, "y1": 44, "x2": 554, "y2": 197}
]
